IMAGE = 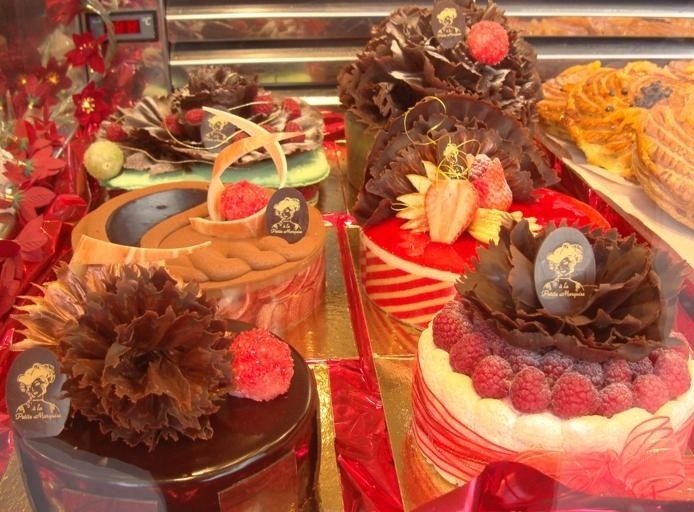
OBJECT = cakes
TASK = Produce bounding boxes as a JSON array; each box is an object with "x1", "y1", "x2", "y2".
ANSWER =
[
  {"x1": 407, "y1": 416, "x2": 694, "y2": 512},
  {"x1": 348, "y1": 91, "x2": 610, "y2": 332},
  {"x1": 6, "y1": 265, "x2": 322, "y2": 511},
  {"x1": 336, "y1": 1, "x2": 546, "y2": 192},
  {"x1": 72, "y1": 179, "x2": 326, "y2": 343},
  {"x1": 413, "y1": 217, "x2": 693, "y2": 484},
  {"x1": 83, "y1": 64, "x2": 324, "y2": 179}
]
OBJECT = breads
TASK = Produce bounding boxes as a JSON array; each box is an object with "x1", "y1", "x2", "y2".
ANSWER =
[
  {"x1": 577, "y1": 59, "x2": 658, "y2": 185},
  {"x1": 627, "y1": 60, "x2": 694, "y2": 233},
  {"x1": 538, "y1": 56, "x2": 612, "y2": 125}
]
